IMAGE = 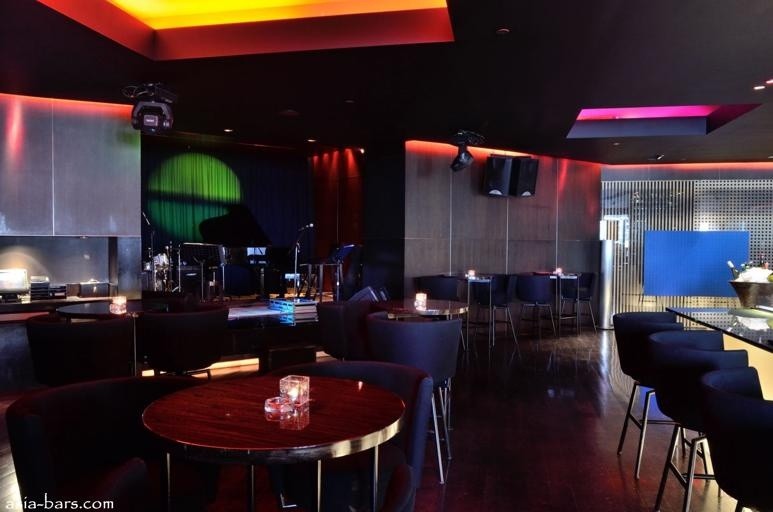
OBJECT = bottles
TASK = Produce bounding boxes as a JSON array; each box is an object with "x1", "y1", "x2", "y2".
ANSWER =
[{"x1": 726, "y1": 254, "x2": 770, "y2": 280}]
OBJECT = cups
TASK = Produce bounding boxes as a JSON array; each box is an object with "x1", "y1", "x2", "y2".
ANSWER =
[
  {"x1": 416, "y1": 292, "x2": 427, "y2": 308},
  {"x1": 555, "y1": 267, "x2": 564, "y2": 276},
  {"x1": 467, "y1": 269, "x2": 476, "y2": 278},
  {"x1": 112, "y1": 295, "x2": 127, "y2": 314},
  {"x1": 279, "y1": 374, "x2": 310, "y2": 432}
]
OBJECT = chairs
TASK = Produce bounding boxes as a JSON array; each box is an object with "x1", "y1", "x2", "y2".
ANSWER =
[
  {"x1": 614, "y1": 313, "x2": 773, "y2": 512},
  {"x1": 417, "y1": 272, "x2": 597, "y2": 351}
]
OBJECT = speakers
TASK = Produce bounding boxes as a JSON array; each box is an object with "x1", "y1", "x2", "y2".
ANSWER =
[
  {"x1": 509, "y1": 156, "x2": 538, "y2": 197},
  {"x1": 349, "y1": 286, "x2": 390, "y2": 302},
  {"x1": 483, "y1": 154, "x2": 512, "y2": 197}
]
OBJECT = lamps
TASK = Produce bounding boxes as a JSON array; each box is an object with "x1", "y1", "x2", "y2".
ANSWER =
[
  {"x1": 451, "y1": 129, "x2": 488, "y2": 172},
  {"x1": 122, "y1": 82, "x2": 179, "y2": 138}
]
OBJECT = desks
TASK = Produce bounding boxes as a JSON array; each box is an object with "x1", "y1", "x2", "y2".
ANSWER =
[
  {"x1": 56, "y1": 301, "x2": 168, "y2": 328},
  {"x1": 141, "y1": 374, "x2": 406, "y2": 512},
  {"x1": 370, "y1": 299, "x2": 469, "y2": 321}
]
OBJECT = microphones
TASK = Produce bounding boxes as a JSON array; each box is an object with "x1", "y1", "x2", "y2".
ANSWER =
[
  {"x1": 142, "y1": 211, "x2": 151, "y2": 226},
  {"x1": 298, "y1": 223, "x2": 314, "y2": 230}
]
